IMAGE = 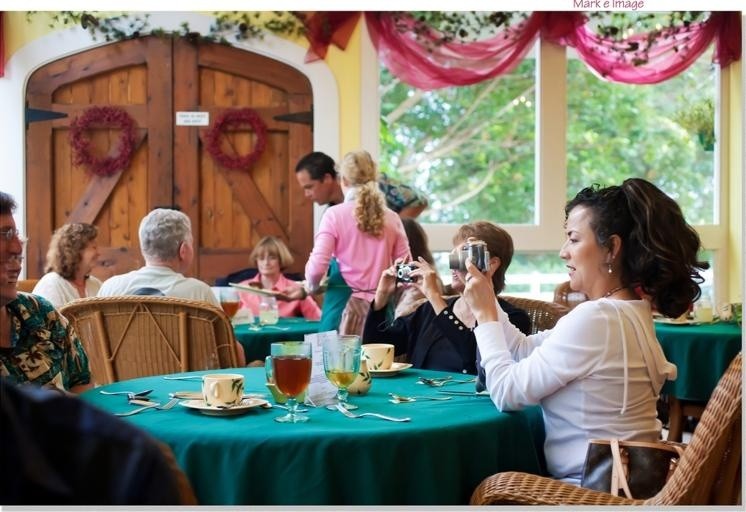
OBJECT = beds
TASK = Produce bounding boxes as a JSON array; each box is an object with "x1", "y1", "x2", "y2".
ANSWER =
[{"x1": 74, "y1": 365, "x2": 546, "y2": 506}]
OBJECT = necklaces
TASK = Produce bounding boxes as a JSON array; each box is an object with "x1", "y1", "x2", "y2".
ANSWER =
[{"x1": 601, "y1": 285, "x2": 625, "y2": 299}]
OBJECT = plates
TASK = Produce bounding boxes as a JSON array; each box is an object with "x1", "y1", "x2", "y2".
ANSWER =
[
  {"x1": 362, "y1": 362, "x2": 415, "y2": 378},
  {"x1": 179, "y1": 397, "x2": 265, "y2": 416}
]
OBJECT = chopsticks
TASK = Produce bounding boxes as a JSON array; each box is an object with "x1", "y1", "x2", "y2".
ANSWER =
[{"x1": 435, "y1": 386, "x2": 483, "y2": 398}]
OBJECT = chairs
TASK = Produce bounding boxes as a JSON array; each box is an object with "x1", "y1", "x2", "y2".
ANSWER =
[
  {"x1": 58, "y1": 294, "x2": 238, "y2": 386},
  {"x1": 554, "y1": 280, "x2": 587, "y2": 311},
  {"x1": 214, "y1": 267, "x2": 303, "y2": 288},
  {"x1": 467, "y1": 352, "x2": 742, "y2": 506},
  {"x1": 501, "y1": 295, "x2": 565, "y2": 337}
]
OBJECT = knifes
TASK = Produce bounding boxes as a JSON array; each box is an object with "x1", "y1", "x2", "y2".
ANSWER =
[{"x1": 168, "y1": 391, "x2": 204, "y2": 401}]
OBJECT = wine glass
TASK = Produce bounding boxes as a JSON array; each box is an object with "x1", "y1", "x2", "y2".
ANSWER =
[
  {"x1": 217, "y1": 286, "x2": 241, "y2": 325},
  {"x1": 322, "y1": 333, "x2": 361, "y2": 412},
  {"x1": 269, "y1": 340, "x2": 313, "y2": 422}
]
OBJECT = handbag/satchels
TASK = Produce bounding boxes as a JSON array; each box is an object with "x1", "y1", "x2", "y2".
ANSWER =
[{"x1": 578, "y1": 436, "x2": 688, "y2": 501}]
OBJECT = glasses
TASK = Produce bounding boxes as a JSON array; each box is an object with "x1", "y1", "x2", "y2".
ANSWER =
[{"x1": 1, "y1": 228, "x2": 21, "y2": 241}]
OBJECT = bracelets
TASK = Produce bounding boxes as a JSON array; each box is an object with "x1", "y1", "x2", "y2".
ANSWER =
[{"x1": 301, "y1": 286, "x2": 307, "y2": 300}]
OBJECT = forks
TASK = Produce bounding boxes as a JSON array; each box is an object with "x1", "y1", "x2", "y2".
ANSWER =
[
  {"x1": 99, "y1": 387, "x2": 154, "y2": 396},
  {"x1": 419, "y1": 375, "x2": 480, "y2": 387},
  {"x1": 113, "y1": 397, "x2": 181, "y2": 416},
  {"x1": 335, "y1": 403, "x2": 408, "y2": 424}
]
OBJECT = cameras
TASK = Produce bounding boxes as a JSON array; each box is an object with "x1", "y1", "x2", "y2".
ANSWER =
[
  {"x1": 448, "y1": 238, "x2": 490, "y2": 273},
  {"x1": 396, "y1": 263, "x2": 417, "y2": 283}
]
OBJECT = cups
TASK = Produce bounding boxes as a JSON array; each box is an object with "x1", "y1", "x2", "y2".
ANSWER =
[
  {"x1": 200, "y1": 373, "x2": 245, "y2": 406},
  {"x1": 257, "y1": 292, "x2": 281, "y2": 324},
  {"x1": 363, "y1": 343, "x2": 397, "y2": 370},
  {"x1": 266, "y1": 381, "x2": 305, "y2": 404},
  {"x1": 264, "y1": 357, "x2": 276, "y2": 382},
  {"x1": 348, "y1": 366, "x2": 372, "y2": 394}
]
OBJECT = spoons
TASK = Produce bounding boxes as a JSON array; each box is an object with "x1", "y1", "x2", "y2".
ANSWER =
[{"x1": 391, "y1": 393, "x2": 451, "y2": 402}]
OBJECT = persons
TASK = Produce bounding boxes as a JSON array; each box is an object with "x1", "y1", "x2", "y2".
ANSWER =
[
  {"x1": 0, "y1": 191, "x2": 22, "y2": 266},
  {"x1": 0, "y1": 238, "x2": 95, "y2": 395},
  {"x1": 32, "y1": 220, "x2": 104, "y2": 311},
  {"x1": 361, "y1": 222, "x2": 530, "y2": 377},
  {"x1": 232, "y1": 236, "x2": 322, "y2": 322},
  {"x1": 401, "y1": 219, "x2": 434, "y2": 267},
  {"x1": 274, "y1": 151, "x2": 435, "y2": 304},
  {"x1": 452, "y1": 177, "x2": 711, "y2": 489},
  {"x1": 303, "y1": 149, "x2": 414, "y2": 338},
  {"x1": 93, "y1": 206, "x2": 265, "y2": 368}
]
